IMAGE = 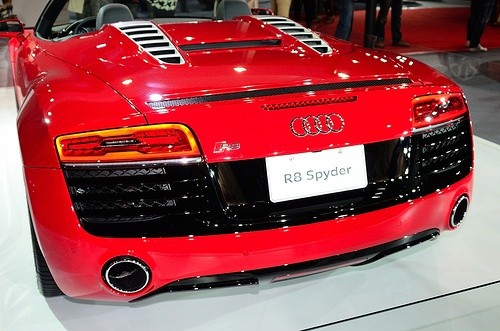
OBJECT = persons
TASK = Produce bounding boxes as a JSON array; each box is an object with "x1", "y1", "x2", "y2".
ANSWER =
[
  {"x1": 466, "y1": 0, "x2": 497, "y2": 52},
  {"x1": 335, "y1": 0, "x2": 375, "y2": 50},
  {"x1": 375, "y1": 0, "x2": 410, "y2": 48},
  {"x1": 292, "y1": 0, "x2": 314, "y2": 31}
]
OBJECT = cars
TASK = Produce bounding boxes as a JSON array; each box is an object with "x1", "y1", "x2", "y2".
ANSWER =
[{"x1": 0, "y1": 0, "x2": 475, "y2": 304}]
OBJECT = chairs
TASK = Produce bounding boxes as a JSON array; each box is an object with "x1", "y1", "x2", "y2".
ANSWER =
[
  {"x1": 95, "y1": 2, "x2": 134, "y2": 29},
  {"x1": 215, "y1": 0, "x2": 253, "y2": 22}
]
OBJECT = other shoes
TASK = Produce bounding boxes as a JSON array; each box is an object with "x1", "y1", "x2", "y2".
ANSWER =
[
  {"x1": 374, "y1": 40, "x2": 385, "y2": 49},
  {"x1": 393, "y1": 41, "x2": 409, "y2": 47},
  {"x1": 466, "y1": 40, "x2": 488, "y2": 53}
]
